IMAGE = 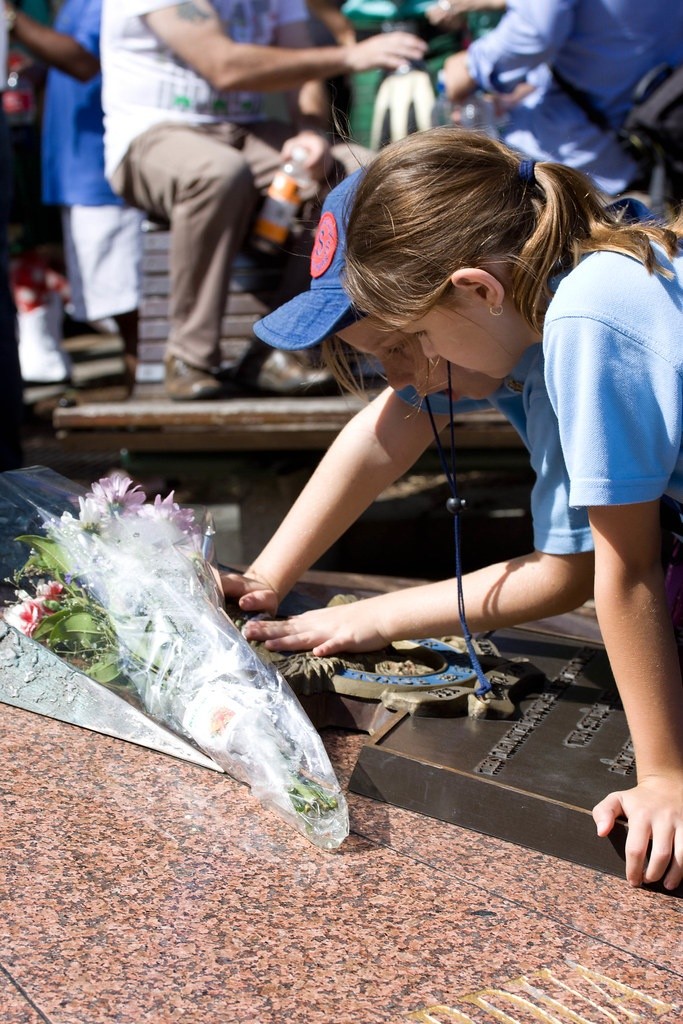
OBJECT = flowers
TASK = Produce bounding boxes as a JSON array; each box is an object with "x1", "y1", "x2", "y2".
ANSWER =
[{"x1": 2, "y1": 473, "x2": 348, "y2": 850}]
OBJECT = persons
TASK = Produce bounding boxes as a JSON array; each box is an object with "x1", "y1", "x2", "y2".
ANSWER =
[
  {"x1": 217, "y1": 126, "x2": 683, "y2": 890},
  {"x1": 0, "y1": 0, "x2": 683, "y2": 398}
]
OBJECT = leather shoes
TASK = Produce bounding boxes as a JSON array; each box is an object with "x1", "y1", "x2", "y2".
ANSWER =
[
  {"x1": 239, "y1": 338, "x2": 333, "y2": 394},
  {"x1": 165, "y1": 355, "x2": 222, "y2": 399}
]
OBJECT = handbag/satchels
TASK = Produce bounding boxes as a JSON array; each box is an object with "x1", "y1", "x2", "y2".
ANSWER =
[{"x1": 619, "y1": 62, "x2": 683, "y2": 166}]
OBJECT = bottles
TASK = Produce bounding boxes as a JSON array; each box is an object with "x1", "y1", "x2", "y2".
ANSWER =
[
  {"x1": 248, "y1": 146, "x2": 309, "y2": 255},
  {"x1": 1, "y1": 55, "x2": 37, "y2": 126},
  {"x1": 431, "y1": 83, "x2": 450, "y2": 127},
  {"x1": 460, "y1": 92, "x2": 497, "y2": 139}
]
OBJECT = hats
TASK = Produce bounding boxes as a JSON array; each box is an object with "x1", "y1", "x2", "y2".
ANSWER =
[{"x1": 253, "y1": 164, "x2": 368, "y2": 349}]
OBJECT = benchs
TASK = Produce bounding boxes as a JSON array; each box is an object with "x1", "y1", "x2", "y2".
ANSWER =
[{"x1": 53, "y1": 230, "x2": 530, "y2": 566}]
